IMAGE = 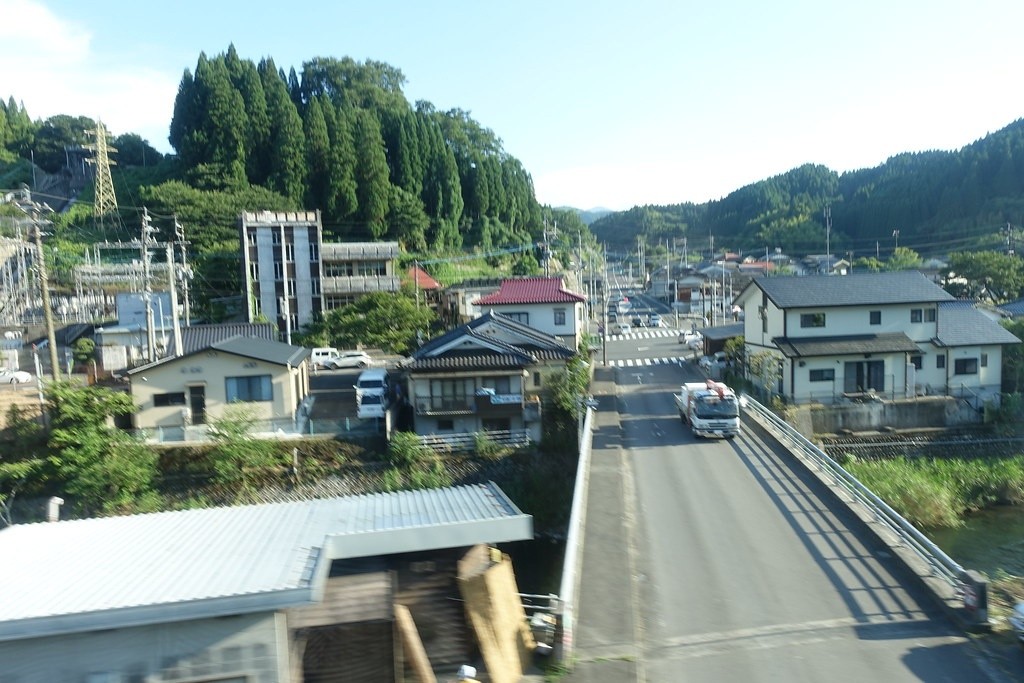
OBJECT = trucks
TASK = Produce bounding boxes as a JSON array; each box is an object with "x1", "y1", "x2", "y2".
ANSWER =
[{"x1": 672, "y1": 378, "x2": 742, "y2": 441}]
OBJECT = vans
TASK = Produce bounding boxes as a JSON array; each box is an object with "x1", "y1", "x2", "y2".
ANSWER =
[
  {"x1": 698, "y1": 350, "x2": 739, "y2": 374},
  {"x1": 310, "y1": 348, "x2": 340, "y2": 366}
]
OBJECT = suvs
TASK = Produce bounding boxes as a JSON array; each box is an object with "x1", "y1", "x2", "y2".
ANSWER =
[{"x1": 324, "y1": 350, "x2": 372, "y2": 369}]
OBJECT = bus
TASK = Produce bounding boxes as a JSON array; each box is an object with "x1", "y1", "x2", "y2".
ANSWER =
[{"x1": 353, "y1": 367, "x2": 389, "y2": 421}]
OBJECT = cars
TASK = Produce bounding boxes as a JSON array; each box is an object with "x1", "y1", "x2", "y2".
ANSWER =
[
  {"x1": 677, "y1": 329, "x2": 704, "y2": 345},
  {"x1": 687, "y1": 335, "x2": 703, "y2": 350},
  {"x1": 606, "y1": 288, "x2": 662, "y2": 335},
  {"x1": 0, "y1": 367, "x2": 31, "y2": 385}
]
